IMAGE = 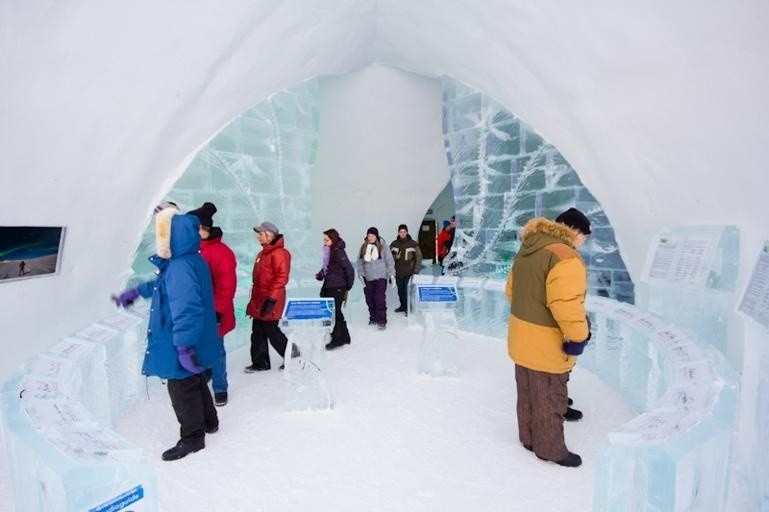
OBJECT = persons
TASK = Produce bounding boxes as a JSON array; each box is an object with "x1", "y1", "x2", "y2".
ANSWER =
[
  {"x1": 183, "y1": 202, "x2": 237, "y2": 408},
  {"x1": 358, "y1": 228, "x2": 397, "y2": 329},
  {"x1": 246, "y1": 220, "x2": 301, "y2": 373},
  {"x1": 435, "y1": 220, "x2": 451, "y2": 275},
  {"x1": 449, "y1": 215, "x2": 456, "y2": 251},
  {"x1": 390, "y1": 225, "x2": 423, "y2": 315},
  {"x1": 17, "y1": 260, "x2": 26, "y2": 277},
  {"x1": 502, "y1": 204, "x2": 592, "y2": 471},
  {"x1": 315, "y1": 228, "x2": 355, "y2": 350},
  {"x1": 563, "y1": 395, "x2": 584, "y2": 422},
  {"x1": 110, "y1": 207, "x2": 223, "y2": 462}
]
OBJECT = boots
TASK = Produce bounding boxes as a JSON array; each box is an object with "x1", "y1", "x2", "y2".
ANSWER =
[{"x1": 326, "y1": 321, "x2": 350, "y2": 348}]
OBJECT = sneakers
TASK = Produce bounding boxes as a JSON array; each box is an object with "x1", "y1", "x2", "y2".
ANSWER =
[
  {"x1": 368, "y1": 317, "x2": 377, "y2": 328},
  {"x1": 215, "y1": 392, "x2": 228, "y2": 405},
  {"x1": 377, "y1": 323, "x2": 385, "y2": 331},
  {"x1": 394, "y1": 306, "x2": 406, "y2": 312},
  {"x1": 567, "y1": 399, "x2": 572, "y2": 405},
  {"x1": 536, "y1": 452, "x2": 582, "y2": 467},
  {"x1": 244, "y1": 364, "x2": 260, "y2": 373},
  {"x1": 562, "y1": 408, "x2": 582, "y2": 421}
]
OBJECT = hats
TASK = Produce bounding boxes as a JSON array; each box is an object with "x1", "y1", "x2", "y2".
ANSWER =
[
  {"x1": 398, "y1": 224, "x2": 407, "y2": 232},
  {"x1": 253, "y1": 221, "x2": 278, "y2": 237},
  {"x1": 367, "y1": 227, "x2": 378, "y2": 238},
  {"x1": 186, "y1": 203, "x2": 216, "y2": 229},
  {"x1": 444, "y1": 221, "x2": 450, "y2": 228},
  {"x1": 154, "y1": 202, "x2": 180, "y2": 215},
  {"x1": 555, "y1": 208, "x2": 590, "y2": 234}
]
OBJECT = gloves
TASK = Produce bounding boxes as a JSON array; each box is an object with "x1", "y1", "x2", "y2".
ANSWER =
[
  {"x1": 316, "y1": 272, "x2": 323, "y2": 280},
  {"x1": 389, "y1": 275, "x2": 396, "y2": 289},
  {"x1": 112, "y1": 288, "x2": 140, "y2": 308},
  {"x1": 359, "y1": 275, "x2": 367, "y2": 288},
  {"x1": 563, "y1": 341, "x2": 584, "y2": 354},
  {"x1": 261, "y1": 299, "x2": 275, "y2": 317},
  {"x1": 177, "y1": 346, "x2": 205, "y2": 375},
  {"x1": 413, "y1": 268, "x2": 419, "y2": 274}
]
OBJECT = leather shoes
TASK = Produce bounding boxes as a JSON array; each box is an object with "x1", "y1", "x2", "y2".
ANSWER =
[{"x1": 161, "y1": 440, "x2": 205, "y2": 461}]
